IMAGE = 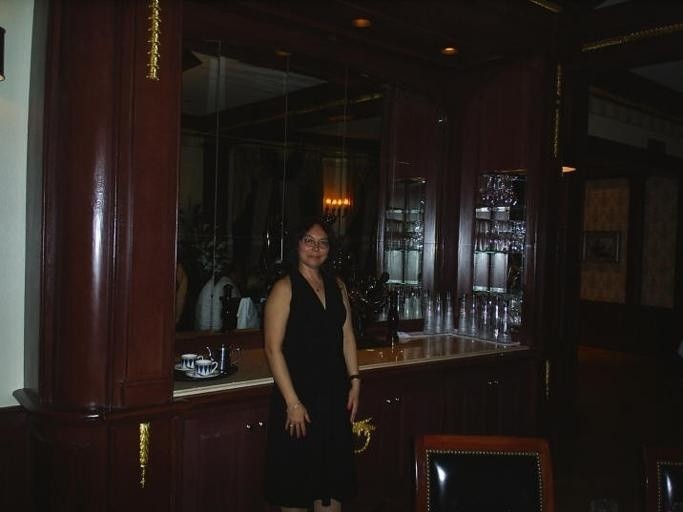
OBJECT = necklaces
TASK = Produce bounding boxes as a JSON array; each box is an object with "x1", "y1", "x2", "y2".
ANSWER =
[{"x1": 303, "y1": 270, "x2": 321, "y2": 292}]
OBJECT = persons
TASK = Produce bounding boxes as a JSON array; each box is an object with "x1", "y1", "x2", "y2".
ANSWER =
[{"x1": 263, "y1": 218, "x2": 360, "y2": 512}]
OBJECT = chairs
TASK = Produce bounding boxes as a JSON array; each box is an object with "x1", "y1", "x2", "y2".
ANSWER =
[
  {"x1": 414, "y1": 431, "x2": 557, "y2": 512},
  {"x1": 640, "y1": 443, "x2": 683, "y2": 512}
]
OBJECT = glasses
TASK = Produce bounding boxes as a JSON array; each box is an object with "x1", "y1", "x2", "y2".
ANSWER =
[{"x1": 304, "y1": 238, "x2": 329, "y2": 249}]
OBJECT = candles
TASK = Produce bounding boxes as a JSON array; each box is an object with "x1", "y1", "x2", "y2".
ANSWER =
[
  {"x1": 326, "y1": 203, "x2": 330, "y2": 210},
  {"x1": 338, "y1": 205, "x2": 341, "y2": 212},
  {"x1": 344, "y1": 203, "x2": 349, "y2": 210},
  {"x1": 333, "y1": 205, "x2": 337, "y2": 212}
]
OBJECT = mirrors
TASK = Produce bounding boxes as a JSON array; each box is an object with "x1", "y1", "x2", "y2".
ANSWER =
[{"x1": 176, "y1": 37, "x2": 449, "y2": 354}]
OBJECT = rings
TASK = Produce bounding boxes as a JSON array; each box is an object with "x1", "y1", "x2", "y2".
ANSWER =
[{"x1": 289, "y1": 425, "x2": 294, "y2": 428}]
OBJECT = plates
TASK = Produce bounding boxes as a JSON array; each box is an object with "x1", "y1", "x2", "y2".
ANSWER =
[
  {"x1": 175, "y1": 363, "x2": 198, "y2": 372},
  {"x1": 185, "y1": 370, "x2": 220, "y2": 379}
]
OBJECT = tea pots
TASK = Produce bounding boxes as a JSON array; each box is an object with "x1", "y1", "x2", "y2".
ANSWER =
[{"x1": 204, "y1": 343, "x2": 241, "y2": 374}]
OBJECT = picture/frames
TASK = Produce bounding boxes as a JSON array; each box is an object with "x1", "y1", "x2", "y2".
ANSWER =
[{"x1": 582, "y1": 230, "x2": 622, "y2": 265}]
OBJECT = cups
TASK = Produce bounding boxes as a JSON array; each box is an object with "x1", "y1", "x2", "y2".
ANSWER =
[
  {"x1": 179, "y1": 353, "x2": 202, "y2": 369},
  {"x1": 195, "y1": 359, "x2": 218, "y2": 375}
]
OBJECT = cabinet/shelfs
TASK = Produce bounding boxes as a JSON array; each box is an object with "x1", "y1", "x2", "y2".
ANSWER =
[
  {"x1": 352, "y1": 372, "x2": 445, "y2": 512},
  {"x1": 182, "y1": 395, "x2": 271, "y2": 512},
  {"x1": 446, "y1": 364, "x2": 531, "y2": 436}
]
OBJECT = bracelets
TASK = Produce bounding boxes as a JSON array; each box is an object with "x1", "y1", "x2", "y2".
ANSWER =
[
  {"x1": 286, "y1": 401, "x2": 302, "y2": 412},
  {"x1": 349, "y1": 374, "x2": 362, "y2": 381}
]
